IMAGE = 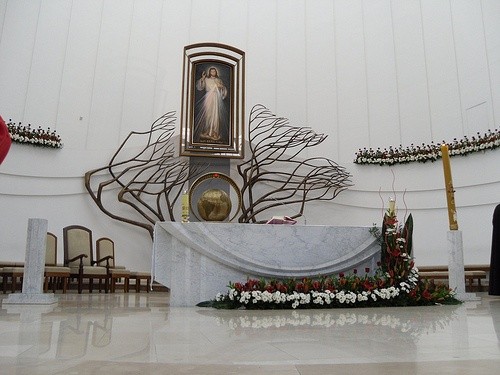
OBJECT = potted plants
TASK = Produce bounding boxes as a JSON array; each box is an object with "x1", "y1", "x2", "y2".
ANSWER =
[{"x1": 441, "y1": 143, "x2": 459, "y2": 230}]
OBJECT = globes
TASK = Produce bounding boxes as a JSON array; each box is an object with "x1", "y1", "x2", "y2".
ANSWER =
[{"x1": 198, "y1": 189, "x2": 232, "y2": 223}]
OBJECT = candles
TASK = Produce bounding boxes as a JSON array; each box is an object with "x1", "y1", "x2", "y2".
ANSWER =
[
  {"x1": 180, "y1": 189, "x2": 190, "y2": 223},
  {"x1": 389, "y1": 197, "x2": 396, "y2": 214}
]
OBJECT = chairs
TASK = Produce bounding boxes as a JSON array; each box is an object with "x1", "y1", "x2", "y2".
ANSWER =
[
  {"x1": 44, "y1": 233, "x2": 70, "y2": 293},
  {"x1": 62, "y1": 226, "x2": 114, "y2": 294},
  {"x1": 96, "y1": 238, "x2": 130, "y2": 294}
]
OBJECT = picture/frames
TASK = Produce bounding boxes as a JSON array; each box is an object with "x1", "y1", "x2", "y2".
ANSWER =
[{"x1": 190, "y1": 59, "x2": 235, "y2": 149}]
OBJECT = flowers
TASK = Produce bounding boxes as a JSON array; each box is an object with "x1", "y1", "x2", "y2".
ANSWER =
[
  {"x1": 212, "y1": 224, "x2": 421, "y2": 304},
  {"x1": 6, "y1": 117, "x2": 64, "y2": 148},
  {"x1": 353, "y1": 127, "x2": 500, "y2": 165}
]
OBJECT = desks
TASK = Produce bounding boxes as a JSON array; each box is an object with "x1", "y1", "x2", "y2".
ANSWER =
[{"x1": 152, "y1": 221, "x2": 413, "y2": 309}]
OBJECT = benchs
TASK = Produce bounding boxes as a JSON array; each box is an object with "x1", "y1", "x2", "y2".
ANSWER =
[
  {"x1": 414, "y1": 263, "x2": 490, "y2": 283},
  {"x1": 417, "y1": 271, "x2": 486, "y2": 291}
]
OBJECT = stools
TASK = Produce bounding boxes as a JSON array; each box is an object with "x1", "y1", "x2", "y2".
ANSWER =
[
  {"x1": 0, "y1": 260, "x2": 25, "y2": 268},
  {"x1": 129, "y1": 271, "x2": 152, "y2": 294},
  {"x1": 0, "y1": 266, "x2": 24, "y2": 294}
]
